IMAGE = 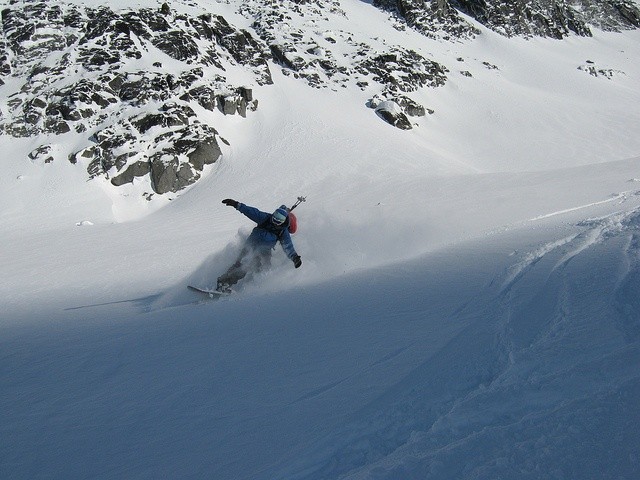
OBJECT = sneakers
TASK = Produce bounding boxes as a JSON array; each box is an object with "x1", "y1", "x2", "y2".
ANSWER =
[{"x1": 217, "y1": 280, "x2": 233, "y2": 293}]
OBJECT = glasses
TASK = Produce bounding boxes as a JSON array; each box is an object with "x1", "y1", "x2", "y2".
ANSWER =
[{"x1": 272, "y1": 215, "x2": 285, "y2": 226}]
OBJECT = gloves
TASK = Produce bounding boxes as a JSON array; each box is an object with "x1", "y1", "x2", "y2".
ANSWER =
[
  {"x1": 292, "y1": 254, "x2": 302, "y2": 269},
  {"x1": 222, "y1": 199, "x2": 240, "y2": 210}
]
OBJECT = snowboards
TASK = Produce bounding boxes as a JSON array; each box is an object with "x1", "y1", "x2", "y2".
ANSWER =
[{"x1": 187, "y1": 286, "x2": 229, "y2": 296}]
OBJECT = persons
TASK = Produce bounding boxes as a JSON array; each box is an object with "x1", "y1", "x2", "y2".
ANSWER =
[{"x1": 215, "y1": 197, "x2": 302, "y2": 293}]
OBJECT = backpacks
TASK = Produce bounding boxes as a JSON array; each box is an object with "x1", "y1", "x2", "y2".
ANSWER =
[{"x1": 254, "y1": 213, "x2": 297, "y2": 252}]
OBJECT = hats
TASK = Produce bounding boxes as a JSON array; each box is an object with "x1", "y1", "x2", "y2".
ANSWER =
[{"x1": 271, "y1": 208, "x2": 287, "y2": 227}]
